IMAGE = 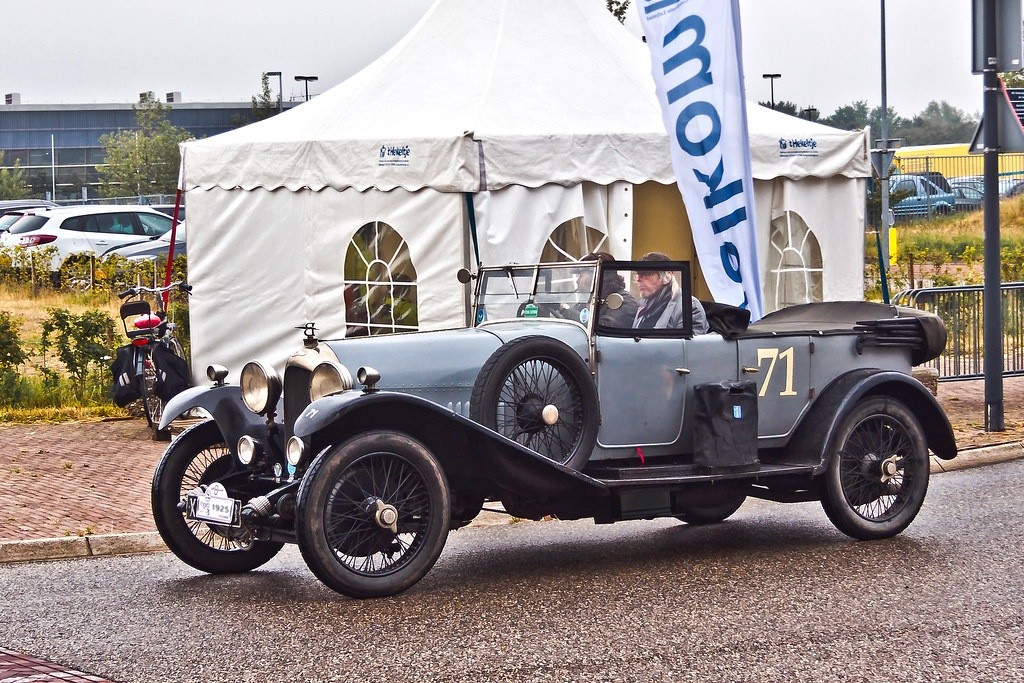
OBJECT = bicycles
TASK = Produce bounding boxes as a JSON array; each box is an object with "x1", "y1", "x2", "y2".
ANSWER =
[{"x1": 111, "y1": 279, "x2": 193, "y2": 433}]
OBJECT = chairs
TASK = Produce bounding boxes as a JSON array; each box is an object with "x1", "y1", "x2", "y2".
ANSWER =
[{"x1": 700, "y1": 300, "x2": 751, "y2": 341}]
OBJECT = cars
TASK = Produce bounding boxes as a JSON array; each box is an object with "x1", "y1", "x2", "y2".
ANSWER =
[
  {"x1": 150, "y1": 262, "x2": 957, "y2": 599},
  {"x1": 956, "y1": 186, "x2": 986, "y2": 212},
  {"x1": 887, "y1": 176, "x2": 955, "y2": 222},
  {"x1": 5, "y1": 205, "x2": 186, "y2": 291},
  {"x1": 997, "y1": 181, "x2": 1022, "y2": 198},
  {"x1": 1, "y1": 200, "x2": 52, "y2": 226}
]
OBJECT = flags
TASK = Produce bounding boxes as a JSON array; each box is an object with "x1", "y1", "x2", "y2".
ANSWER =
[{"x1": 638, "y1": 0, "x2": 763, "y2": 324}]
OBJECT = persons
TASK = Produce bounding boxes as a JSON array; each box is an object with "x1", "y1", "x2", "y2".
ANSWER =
[
  {"x1": 632, "y1": 251, "x2": 710, "y2": 333},
  {"x1": 537, "y1": 252, "x2": 640, "y2": 329}
]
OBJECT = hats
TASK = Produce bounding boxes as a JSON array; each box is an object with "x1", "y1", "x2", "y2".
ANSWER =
[
  {"x1": 637, "y1": 252, "x2": 672, "y2": 261},
  {"x1": 569, "y1": 252, "x2": 617, "y2": 274}
]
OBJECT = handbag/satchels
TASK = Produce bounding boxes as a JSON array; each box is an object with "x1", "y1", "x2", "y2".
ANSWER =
[
  {"x1": 151, "y1": 343, "x2": 190, "y2": 402},
  {"x1": 110, "y1": 344, "x2": 145, "y2": 408}
]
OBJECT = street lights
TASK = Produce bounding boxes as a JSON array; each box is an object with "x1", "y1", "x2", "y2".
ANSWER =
[
  {"x1": 762, "y1": 73, "x2": 781, "y2": 110},
  {"x1": 804, "y1": 106, "x2": 817, "y2": 121},
  {"x1": 294, "y1": 76, "x2": 318, "y2": 101},
  {"x1": 266, "y1": 72, "x2": 283, "y2": 113}
]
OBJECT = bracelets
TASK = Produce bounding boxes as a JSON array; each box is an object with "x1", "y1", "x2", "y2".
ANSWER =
[{"x1": 560, "y1": 304, "x2": 570, "y2": 313}]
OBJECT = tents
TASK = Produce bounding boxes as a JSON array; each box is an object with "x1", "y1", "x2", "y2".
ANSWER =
[{"x1": 178, "y1": 0, "x2": 871, "y2": 424}]
